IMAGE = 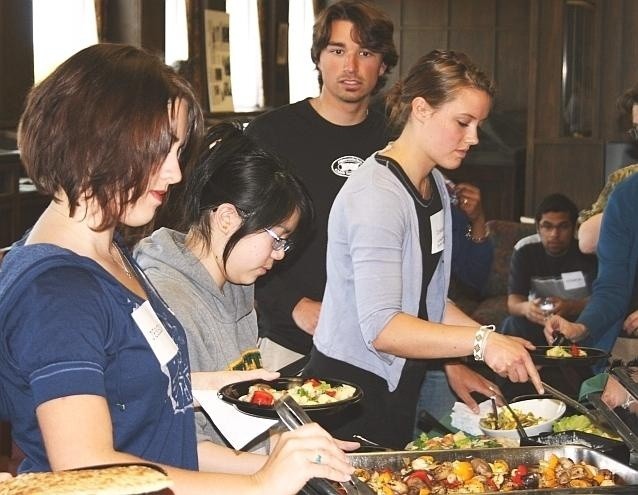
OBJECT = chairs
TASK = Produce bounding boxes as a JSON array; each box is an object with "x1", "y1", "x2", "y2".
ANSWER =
[{"x1": 467, "y1": 219, "x2": 536, "y2": 333}]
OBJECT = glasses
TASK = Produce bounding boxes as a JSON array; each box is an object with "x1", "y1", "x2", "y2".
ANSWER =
[
  {"x1": 541, "y1": 222, "x2": 571, "y2": 233},
  {"x1": 264, "y1": 228, "x2": 295, "y2": 252}
]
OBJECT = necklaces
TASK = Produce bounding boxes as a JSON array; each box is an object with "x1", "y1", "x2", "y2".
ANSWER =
[{"x1": 49, "y1": 206, "x2": 133, "y2": 279}]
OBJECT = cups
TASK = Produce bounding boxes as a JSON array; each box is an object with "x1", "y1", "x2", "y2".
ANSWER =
[
  {"x1": 444, "y1": 181, "x2": 459, "y2": 205},
  {"x1": 539, "y1": 296, "x2": 556, "y2": 318}
]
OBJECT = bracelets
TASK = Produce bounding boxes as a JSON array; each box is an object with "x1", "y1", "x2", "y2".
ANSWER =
[
  {"x1": 474, "y1": 324, "x2": 495, "y2": 362},
  {"x1": 465, "y1": 223, "x2": 490, "y2": 240}
]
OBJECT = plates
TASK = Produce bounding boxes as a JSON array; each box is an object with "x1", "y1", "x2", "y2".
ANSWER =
[
  {"x1": 528, "y1": 345, "x2": 608, "y2": 365},
  {"x1": 216, "y1": 376, "x2": 366, "y2": 420}
]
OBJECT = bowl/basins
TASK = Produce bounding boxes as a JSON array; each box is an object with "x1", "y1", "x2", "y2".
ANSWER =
[
  {"x1": 478, "y1": 398, "x2": 567, "y2": 439},
  {"x1": 520, "y1": 430, "x2": 630, "y2": 466}
]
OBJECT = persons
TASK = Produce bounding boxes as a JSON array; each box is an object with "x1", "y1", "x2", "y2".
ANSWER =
[
  {"x1": 501, "y1": 193, "x2": 599, "y2": 349},
  {"x1": 577, "y1": 163, "x2": 638, "y2": 252},
  {"x1": 295, "y1": 46, "x2": 545, "y2": 453},
  {"x1": 418, "y1": 178, "x2": 494, "y2": 421},
  {"x1": 544, "y1": 85, "x2": 638, "y2": 374},
  {"x1": 238, "y1": 0, "x2": 511, "y2": 421},
  {"x1": 131, "y1": 117, "x2": 318, "y2": 476},
  {"x1": 2, "y1": 41, "x2": 362, "y2": 495}
]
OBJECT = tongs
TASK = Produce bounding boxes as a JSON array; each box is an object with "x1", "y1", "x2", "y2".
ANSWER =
[
  {"x1": 490, "y1": 392, "x2": 528, "y2": 439},
  {"x1": 274, "y1": 391, "x2": 379, "y2": 495}
]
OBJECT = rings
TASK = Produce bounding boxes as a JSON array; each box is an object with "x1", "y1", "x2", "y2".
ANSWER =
[
  {"x1": 464, "y1": 198, "x2": 467, "y2": 204},
  {"x1": 490, "y1": 394, "x2": 497, "y2": 401},
  {"x1": 314, "y1": 453, "x2": 322, "y2": 464},
  {"x1": 489, "y1": 385, "x2": 495, "y2": 392}
]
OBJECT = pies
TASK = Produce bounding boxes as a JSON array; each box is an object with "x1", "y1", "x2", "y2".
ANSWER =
[{"x1": 0, "y1": 466, "x2": 170, "y2": 495}]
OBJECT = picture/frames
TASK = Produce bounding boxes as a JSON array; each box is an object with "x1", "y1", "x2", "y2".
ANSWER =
[{"x1": 202, "y1": 7, "x2": 268, "y2": 118}]
